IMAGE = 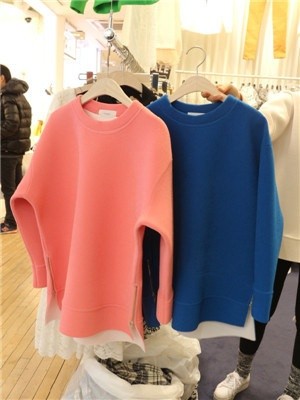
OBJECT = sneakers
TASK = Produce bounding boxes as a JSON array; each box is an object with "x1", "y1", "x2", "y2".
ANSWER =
[
  {"x1": 212, "y1": 370, "x2": 251, "y2": 400},
  {"x1": 0, "y1": 223, "x2": 18, "y2": 234},
  {"x1": 276, "y1": 394, "x2": 294, "y2": 400}
]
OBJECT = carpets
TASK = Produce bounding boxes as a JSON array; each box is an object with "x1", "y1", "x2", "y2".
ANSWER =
[{"x1": 188, "y1": 260, "x2": 299, "y2": 400}]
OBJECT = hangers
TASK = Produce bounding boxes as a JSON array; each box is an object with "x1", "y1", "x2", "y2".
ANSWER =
[{"x1": 74, "y1": 26, "x2": 229, "y2": 108}]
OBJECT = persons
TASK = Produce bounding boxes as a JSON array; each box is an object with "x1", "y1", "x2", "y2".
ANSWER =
[
  {"x1": 0, "y1": 64, "x2": 31, "y2": 234},
  {"x1": 200, "y1": 85, "x2": 300, "y2": 400}
]
